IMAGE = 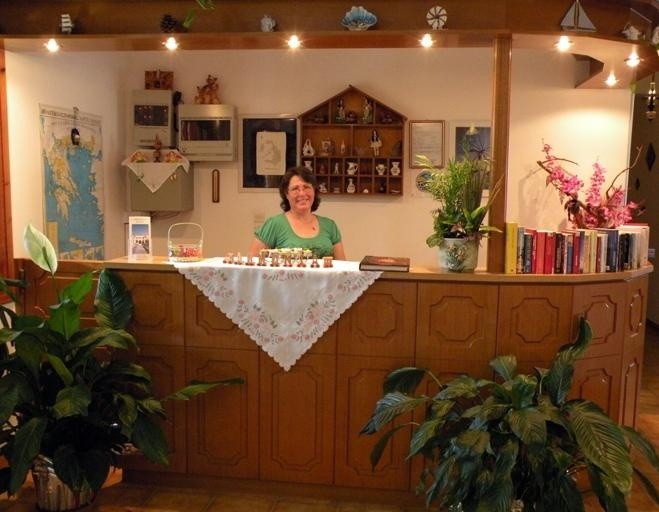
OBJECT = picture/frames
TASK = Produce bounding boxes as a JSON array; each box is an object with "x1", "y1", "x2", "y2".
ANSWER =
[
  {"x1": 238, "y1": 114, "x2": 300, "y2": 192},
  {"x1": 449, "y1": 120, "x2": 493, "y2": 165},
  {"x1": 409, "y1": 120, "x2": 445, "y2": 169}
]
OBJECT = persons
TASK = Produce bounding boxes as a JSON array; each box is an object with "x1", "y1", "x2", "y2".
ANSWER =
[{"x1": 250, "y1": 166, "x2": 348, "y2": 262}]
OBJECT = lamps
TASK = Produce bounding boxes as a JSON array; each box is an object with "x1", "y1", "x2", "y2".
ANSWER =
[{"x1": 646, "y1": 74, "x2": 657, "y2": 123}]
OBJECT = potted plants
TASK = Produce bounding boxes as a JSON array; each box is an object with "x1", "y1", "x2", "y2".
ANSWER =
[{"x1": 0, "y1": 223, "x2": 248, "y2": 512}]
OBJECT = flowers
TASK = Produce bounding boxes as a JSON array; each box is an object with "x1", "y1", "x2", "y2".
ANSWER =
[
  {"x1": 413, "y1": 125, "x2": 504, "y2": 247},
  {"x1": 537, "y1": 139, "x2": 649, "y2": 229}
]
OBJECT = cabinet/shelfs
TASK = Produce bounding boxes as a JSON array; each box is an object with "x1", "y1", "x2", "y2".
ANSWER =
[{"x1": 296, "y1": 84, "x2": 408, "y2": 197}]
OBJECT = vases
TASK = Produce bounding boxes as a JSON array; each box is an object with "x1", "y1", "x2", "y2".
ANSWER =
[{"x1": 436, "y1": 238, "x2": 479, "y2": 273}]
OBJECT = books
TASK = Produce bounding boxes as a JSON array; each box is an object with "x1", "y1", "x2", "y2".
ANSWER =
[
  {"x1": 506, "y1": 220, "x2": 652, "y2": 276},
  {"x1": 359, "y1": 255, "x2": 411, "y2": 272}
]
